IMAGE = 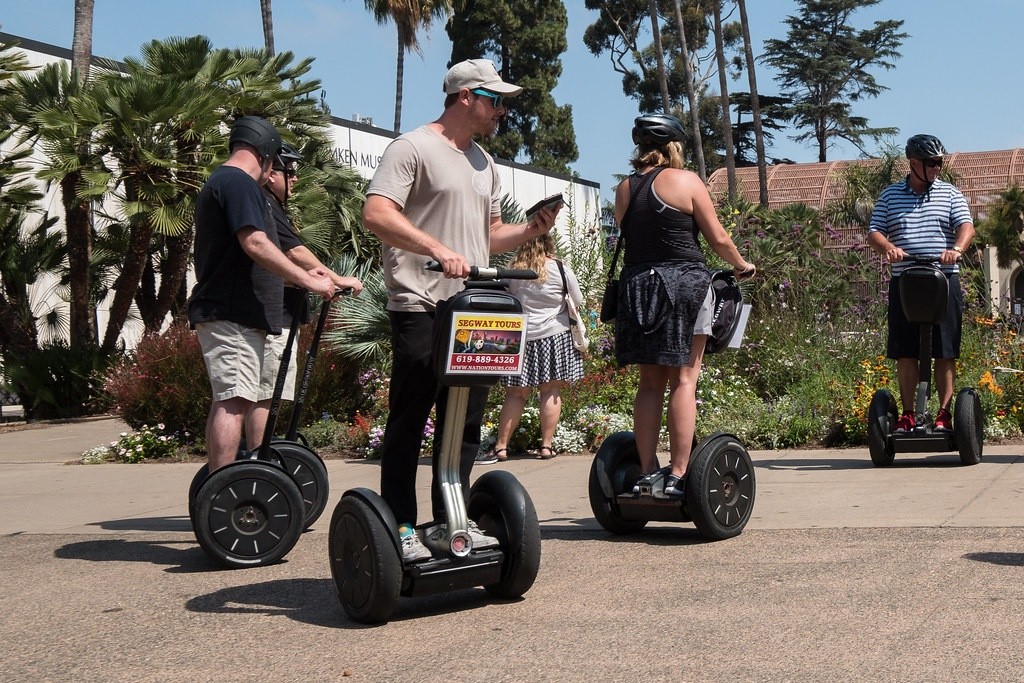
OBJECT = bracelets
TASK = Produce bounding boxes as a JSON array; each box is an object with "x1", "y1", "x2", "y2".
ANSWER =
[{"x1": 735, "y1": 267, "x2": 746, "y2": 272}]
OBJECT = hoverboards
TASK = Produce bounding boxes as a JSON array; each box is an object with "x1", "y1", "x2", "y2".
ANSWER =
[
  {"x1": 188, "y1": 286, "x2": 313, "y2": 571},
  {"x1": 588, "y1": 269, "x2": 756, "y2": 541},
  {"x1": 327, "y1": 260, "x2": 541, "y2": 624},
  {"x1": 238, "y1": 287, "x2": 352, "y2": 532},
  {"x1": 868, "y1": 257, "x2": 984, "y2": 467}
]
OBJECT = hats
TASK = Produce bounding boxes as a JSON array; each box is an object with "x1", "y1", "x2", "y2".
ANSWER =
[{"x1": 444, "y1": 58, "x2": 523, "y2": 96}]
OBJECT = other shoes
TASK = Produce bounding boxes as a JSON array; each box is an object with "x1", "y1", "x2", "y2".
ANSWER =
[
  {"x1": 633, "y1": 474, "x2": 646, "y2": 492},
  {"x1": 665, "y1": 472, "x2": 684, "y2": 495}
]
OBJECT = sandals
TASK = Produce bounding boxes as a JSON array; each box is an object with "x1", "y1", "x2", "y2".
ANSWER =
[
  {"x1": 483, "y1": 447, "x2": 509, "y2": 461},
  {"x1": 540, "y1": 447, "x2": 557, "y2": 459}
]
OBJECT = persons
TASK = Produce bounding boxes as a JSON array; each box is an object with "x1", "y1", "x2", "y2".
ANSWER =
[
  {"x1": 362, "y1": 58, "x2": 561, "y2": 562},
  {"x1": 867, "y1": 134, "x2": 975, "y2": 432},
  {"x1": 186, "y1": 115, "x2": 362, "y2": 474},
  {"x1": 485, "y1": 232, "x2": 584, "y2": 461},
  {"x1": 615, "y1": 113, "x2": 756, "y2": 494}
]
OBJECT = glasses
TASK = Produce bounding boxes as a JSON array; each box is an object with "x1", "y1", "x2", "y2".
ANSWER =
[
  {"x1": 471, "y1": 89, "x2": 503, "y2": 108},
  {"x1": 273, "y1": 168, "x2": 296, "y2": 178},
  {"x1": 915, "y1": 158, "x2": 944, "y2": 167}
]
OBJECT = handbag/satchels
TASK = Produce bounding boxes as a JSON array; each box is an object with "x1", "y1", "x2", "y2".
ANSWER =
[
  {"x1": 599, "y1": 281, "x2": 621, "y2": 323},
  {"x1": 433, "y1": 288, "x2": 523, "y2": 388},
  {"x1": 705, "y1": 269, "x2": 743, "y2": 354},
  {"x1": 554, "y1": 259, "x2": 589, "y2": 352},
  {"x1": 899, "y1": 263, "x2": 948, "y2": 323}
]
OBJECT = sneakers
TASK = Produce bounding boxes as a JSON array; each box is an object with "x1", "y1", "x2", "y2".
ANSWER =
[
  {"x1": 931, "y1": 407, "x2": 953, "y2": 432},
  {"x1": 433, "y1": 522, "x2": 498, "y2": 550},
  {"x1": 396, "y1": 523, "x2": 432, "y2": 564},
  {"x1": 893, "y1": 414, "x2": 914, "y2": 433}
]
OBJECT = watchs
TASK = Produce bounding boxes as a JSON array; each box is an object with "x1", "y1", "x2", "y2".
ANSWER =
[{"x1": 953, "y1": 246, "x2": 963, "y2": 254}]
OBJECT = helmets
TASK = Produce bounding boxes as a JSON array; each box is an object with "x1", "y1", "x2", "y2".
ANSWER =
[
  {"x1": 276, "y1": 142, "x2": 305, "y2": 159},
  {"x1": 632, "y1": 112, "x2": 684, "y2": 146},
  {"x1": 228, "y1": 116, "x2": 283, "y2": 158},
  {"x1": 904, "y1": 133, "x2": 947, "y2": 159}
]
